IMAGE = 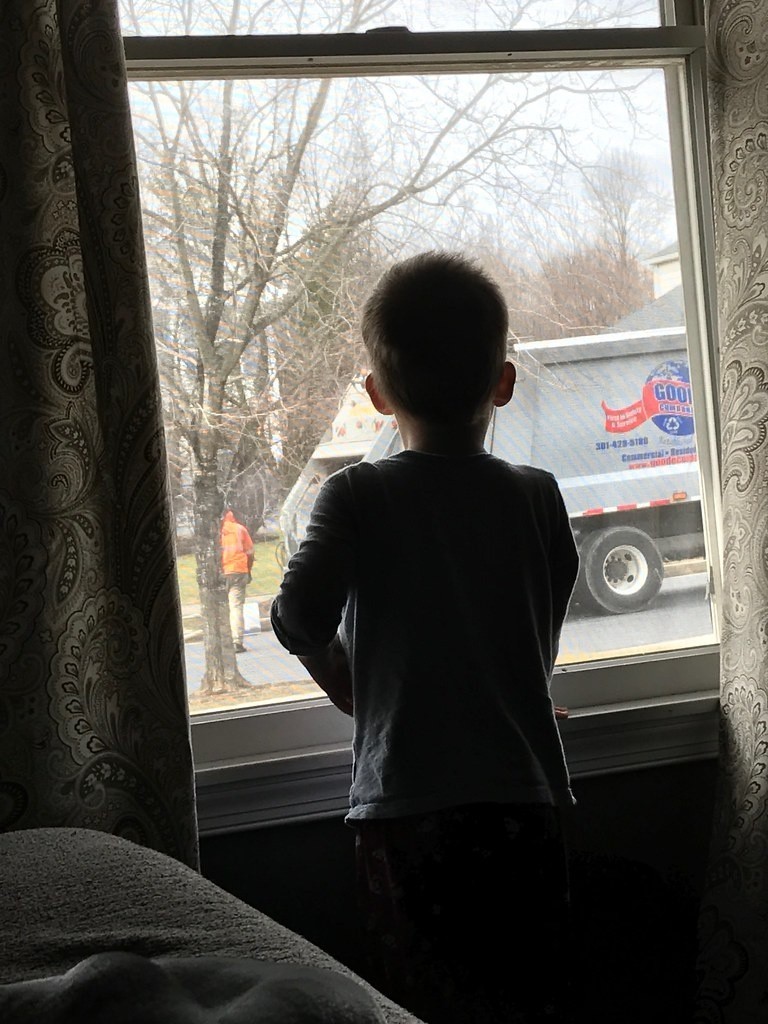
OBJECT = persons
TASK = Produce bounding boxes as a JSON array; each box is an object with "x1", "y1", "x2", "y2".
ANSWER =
[
  {"x1": 220, "y1": 504, "x2": 254, "y2": 653},
  {"x1": 267, "y1": 246, "x2": 581, "y2": 1004}
]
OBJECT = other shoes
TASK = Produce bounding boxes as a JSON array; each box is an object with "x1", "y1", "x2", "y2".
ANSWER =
[{"x1": 232, "y1": 644, "x2": 244, "y2": 652}]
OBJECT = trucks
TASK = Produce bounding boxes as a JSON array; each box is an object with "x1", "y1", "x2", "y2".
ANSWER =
[{"x1": 278, "y1": 325, "x2": 706, "y2": 614}]
